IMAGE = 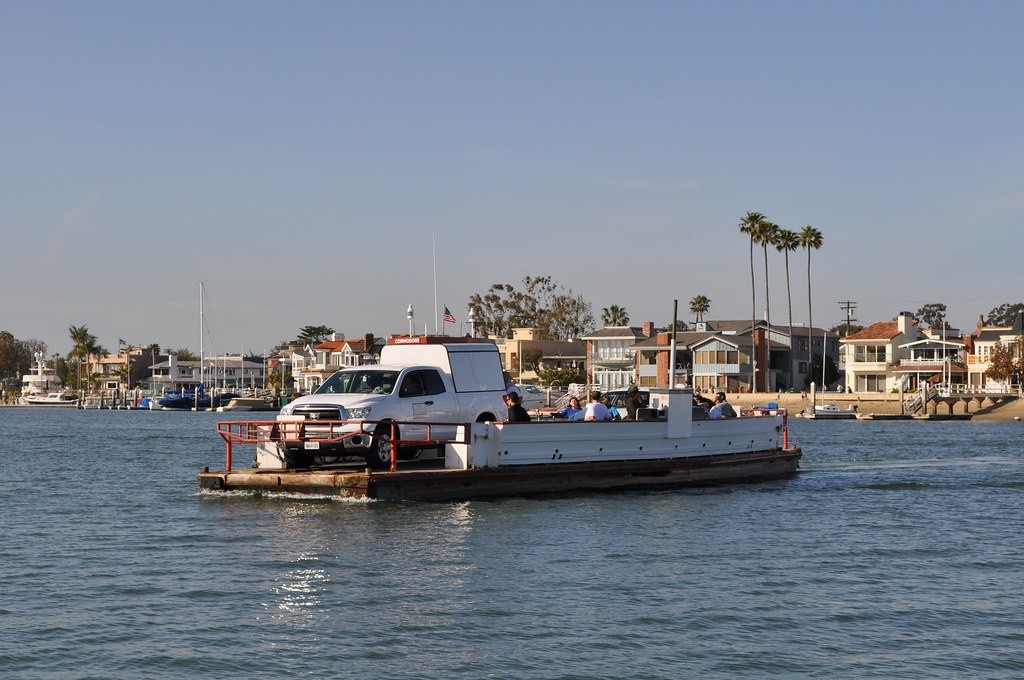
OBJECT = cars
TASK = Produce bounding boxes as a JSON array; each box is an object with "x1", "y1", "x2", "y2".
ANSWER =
[{"x1": 600, "y1": 387, "x2": 649, "y2": 420}]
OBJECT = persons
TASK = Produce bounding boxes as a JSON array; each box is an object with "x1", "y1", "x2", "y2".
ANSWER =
[
  {"x1": 507, "y1": 392, "x2": 531, "y2": 422},
  {"x1": 693, "y1": 392, "x2": 737, "y2": 419},
  {"x1": 626, "y1": 384, "x2": 646, "y2": 420},
  {"x1": 557, "y1": 391, "x2": 621, "y2": 421}
]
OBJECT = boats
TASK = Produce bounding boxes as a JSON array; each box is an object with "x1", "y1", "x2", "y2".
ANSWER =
[
  {"x1": 192, "y1": 230, "x2": 804, "y2": 502},
  {"x1": 514, "y1": 340, "x2": 560, "y2": 413},
  {"x1": 24, "y1": 386, "x2": 79, "y2": 405}
]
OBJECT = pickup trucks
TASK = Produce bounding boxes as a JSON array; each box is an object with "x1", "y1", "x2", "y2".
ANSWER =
[{"x1": 274, "y1": 342, "x2": 511, "y2": 469}]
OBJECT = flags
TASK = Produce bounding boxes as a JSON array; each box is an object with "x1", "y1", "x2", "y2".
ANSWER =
[{"x1": 444, "y1": 307, "x2": 455, "y2": 323}]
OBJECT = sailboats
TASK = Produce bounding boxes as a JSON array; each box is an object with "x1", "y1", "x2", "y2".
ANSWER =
[
  {"x1": 136, "y1": 283, "x2": 241, "y2": 411},
  {"x1": 815, "y1": 332, "x2": 852, "y2": 412}
]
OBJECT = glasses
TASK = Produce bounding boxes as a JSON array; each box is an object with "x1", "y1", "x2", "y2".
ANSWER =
[{"x1": 505, "y1": 398, "x2": 511, "y2": 400}]
